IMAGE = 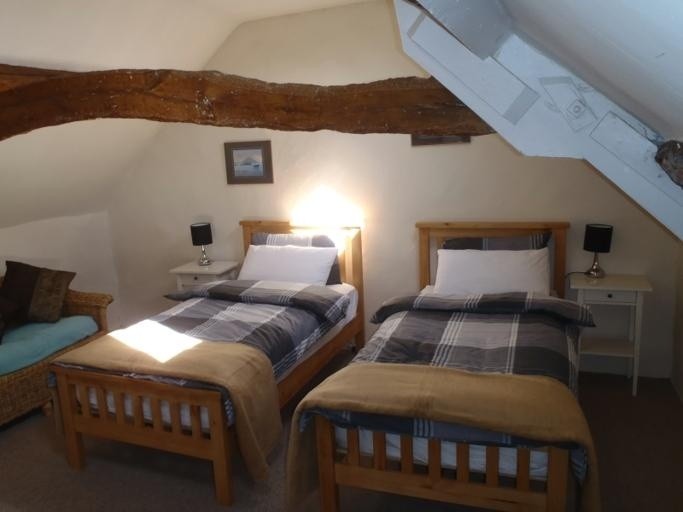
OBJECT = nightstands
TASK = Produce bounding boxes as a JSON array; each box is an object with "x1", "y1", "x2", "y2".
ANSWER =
[
  {"x1": 168, "y1": 260, "x2": 240, "y2": 291},
  {"x1": 568, "y1": 271, "x2": 654, "y2": 397}
]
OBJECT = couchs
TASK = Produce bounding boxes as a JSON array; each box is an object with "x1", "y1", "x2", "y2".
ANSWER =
[{"x1": 0, "y1": 275, "x2": 112, "y2": 426}]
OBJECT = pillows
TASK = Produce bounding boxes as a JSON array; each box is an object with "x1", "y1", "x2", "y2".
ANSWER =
[
  {"x1": 444, "y1": 233, "x2": 555, "y2": 287},
  {"x1": 251, "y1": 232, "x2": 341, "y2": 285},
  {"x1": 434, "y1": 246, "x2": 550, "y2": 297},
  {"x1": 0, "y1": 261, "x2": 76, "y2": 323},
  {"x1": 236, "y1": 243, "x2": 338, "y2": 286}
]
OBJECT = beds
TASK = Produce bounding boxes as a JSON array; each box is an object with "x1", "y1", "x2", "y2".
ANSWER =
[
  {"x1": 47, "y1": 219, "x2": 366, "y2": 507},
  {"x1": 285, "y1": 222, "x2": 601, "y2": 510}
]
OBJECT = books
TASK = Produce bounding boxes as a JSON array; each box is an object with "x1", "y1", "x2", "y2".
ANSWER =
[{"x1": 601, "y1": 334, "x2": 631, "y2": 353}]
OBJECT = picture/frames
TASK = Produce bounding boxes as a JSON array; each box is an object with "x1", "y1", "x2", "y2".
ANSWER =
[{"x1": 224, "y1": 139, "x2": 274, "y2": 184}]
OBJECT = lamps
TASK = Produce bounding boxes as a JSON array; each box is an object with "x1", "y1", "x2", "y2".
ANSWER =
[
  {"x1": 190, "y1": 222, "x2": 213, "y2": 266},
  {"x1": 582, "y1": 224, "x2": 613, "y2": 279}
]
